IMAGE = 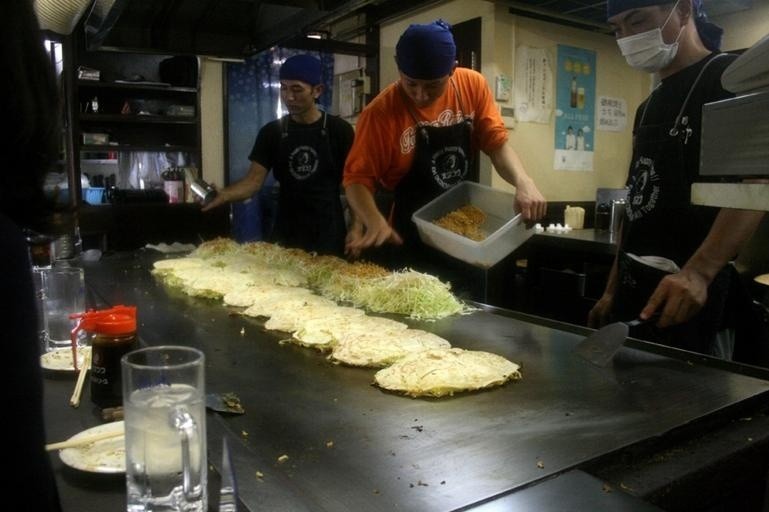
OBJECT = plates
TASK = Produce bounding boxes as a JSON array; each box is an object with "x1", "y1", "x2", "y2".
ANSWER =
[{"x1": 64, "y1": 37, "x2": 232, "y2": 248}]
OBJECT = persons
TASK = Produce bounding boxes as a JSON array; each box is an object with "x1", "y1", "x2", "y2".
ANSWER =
[
  {"x1": 341, "y1": 20, "x2": 548, "y2": 301},
  {"x1": 589, "y1": 0, "x2": 767, "y2": 362},
  {"x1": 196, "y1": 53, "x2": 363, "y2": 263},
  {"x1": 1, "y1": 0, "x2": 61, "y2": 512}
]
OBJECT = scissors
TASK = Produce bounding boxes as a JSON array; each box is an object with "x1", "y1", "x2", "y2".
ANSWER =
[
  {"x1": 575, "y1": 319, "x2": 650, "y2": 367},
  {"x1": 103, "y1": 392, "x2": 245, "y2": 421}
]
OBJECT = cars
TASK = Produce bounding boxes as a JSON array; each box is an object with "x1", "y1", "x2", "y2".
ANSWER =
[
  {"x1": 40, "y1": 266, "x2": 87, "y2": 344},
  {"x1": 121, "y1": 344, "x2": 211, "y2": 511},
  {"x1": 27, "y1": 233, "x2": 54, "y2": 270}
]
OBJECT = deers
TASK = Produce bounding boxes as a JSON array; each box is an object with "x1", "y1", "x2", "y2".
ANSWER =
[{"x1": 616, "y1": 0, "x2": 687, "y2": 75}]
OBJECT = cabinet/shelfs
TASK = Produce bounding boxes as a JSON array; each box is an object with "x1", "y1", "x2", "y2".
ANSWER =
[
  {"x1": 190, "y1": 177, "x2": 217, "y2": 204},
  {"x1": 70, "y1": 302, "x2": 147, "y2": 417},
  {"x1": 563, "y1": 199, "x2": 626, "y2": 234}
]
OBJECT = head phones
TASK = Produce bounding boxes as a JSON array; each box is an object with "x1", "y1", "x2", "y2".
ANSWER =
[
  {"x1": 37, "y1": 428, "x2": 121, "y2": 455},
  {"x1": 69, "y1": 354, "x2": 91, "y2": 408}
]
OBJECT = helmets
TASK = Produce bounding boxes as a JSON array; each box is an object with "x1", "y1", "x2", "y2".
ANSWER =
[
  {"x1": 62, "y1": 421, "x2": 124, "y2": 476},
  {"x1": 40, "y1": 346, "x2": 94, "y2": 372}
]
